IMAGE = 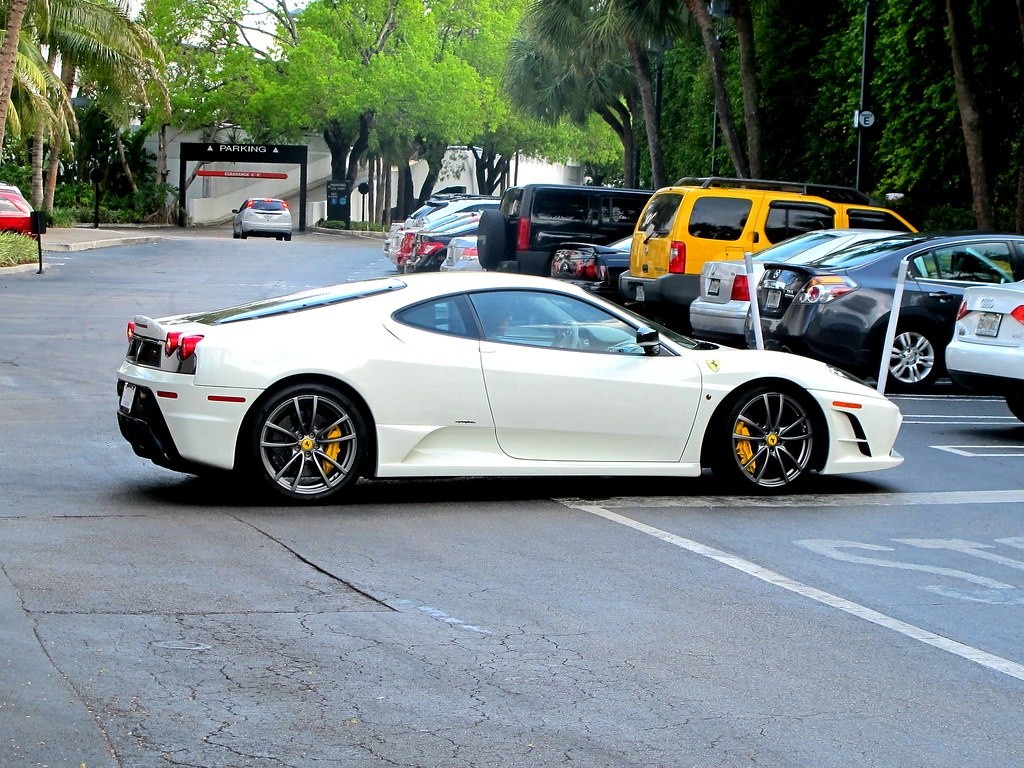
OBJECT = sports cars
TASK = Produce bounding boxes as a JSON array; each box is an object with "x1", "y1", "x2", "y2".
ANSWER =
[{"x1": 119, "y1": 272, "x2": 903, "y2": 508}]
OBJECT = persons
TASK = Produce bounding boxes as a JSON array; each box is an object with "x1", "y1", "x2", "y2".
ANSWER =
[{"x1": 476, "y1": 297, "x2": 509, "y2": 342}]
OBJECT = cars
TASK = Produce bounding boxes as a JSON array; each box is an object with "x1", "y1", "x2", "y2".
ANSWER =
[
  {"x1": 690, "y1": 228, "x2": 928, "y2": 341},
  {"x1": 232, "y1": 197, "x2": 292, "y2": 241},
  {"x1": 0, "y1": 185, "x2": 36, "y2": 239},
  {"x1": 942, "y1": 280, "x2": 1022, "y2": 423},
  {"x1": 382, "y1": 193, "x2": 504, "y2": 274},
  {"x1": 741, "y1": 235, "x2": 1024, "y2": 394},
  {"x1": 550, "y1": 235, "x2": 635, "y2": 300}
]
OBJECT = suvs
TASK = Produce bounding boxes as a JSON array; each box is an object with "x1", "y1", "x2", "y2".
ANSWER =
[
  {"x1": 618, "y1": 177, "x2": 919, "y2": 333},
  {"x1": 476, "y1": 182, "x2": 660, "y2": 276}
]
